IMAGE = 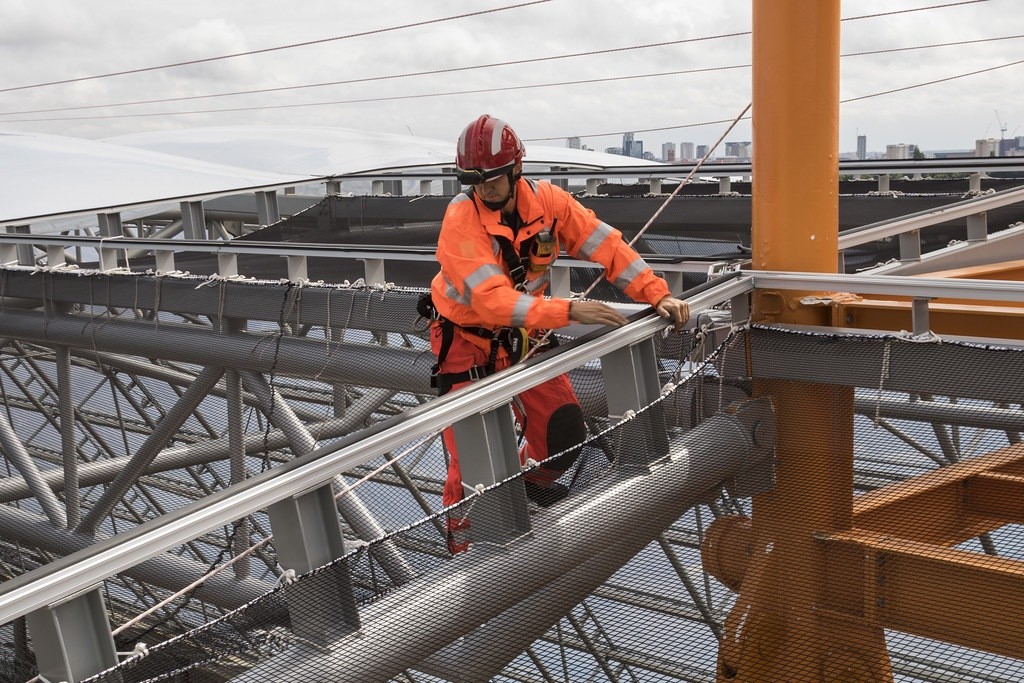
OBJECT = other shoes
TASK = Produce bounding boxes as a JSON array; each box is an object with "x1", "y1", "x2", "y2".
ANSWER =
[{"x1": 525, "y1": 482, "x2": 569, "y2": 508}]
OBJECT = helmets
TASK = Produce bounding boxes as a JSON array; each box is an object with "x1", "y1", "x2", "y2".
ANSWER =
[{"x1": 455, "y1": 114, "x2": 527, "y2": 171}]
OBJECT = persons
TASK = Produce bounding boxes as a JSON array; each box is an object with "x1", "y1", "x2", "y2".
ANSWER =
[{"x1": 427, "y1": 114, "x2": 690, "y2": 552}]
